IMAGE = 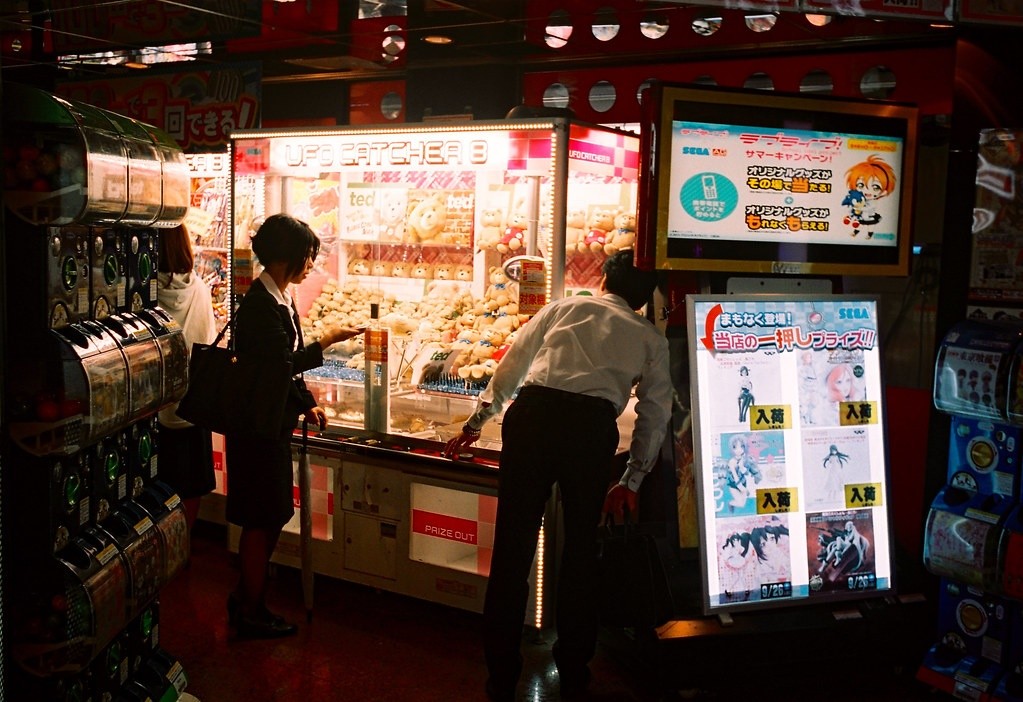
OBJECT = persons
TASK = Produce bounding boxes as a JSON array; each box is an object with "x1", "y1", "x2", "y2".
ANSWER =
[
  {"x1": 154, "y1": 224, "x2": 215, "y2": 527},
  {"x1": 443, "y1": 249, "x2": 672, "y2": 701},
  {"x1": 224, "y1": 214, "x2": 367, "y2": 636}
]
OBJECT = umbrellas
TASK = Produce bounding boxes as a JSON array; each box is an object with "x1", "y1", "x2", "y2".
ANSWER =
[{"x1": 298, "y1": 413, "x2": 327, "y2": 623}]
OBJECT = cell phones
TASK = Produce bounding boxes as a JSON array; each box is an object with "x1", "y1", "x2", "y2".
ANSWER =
[{"x1": 350, "y1": 324, "x2": 369, "y2": 330}]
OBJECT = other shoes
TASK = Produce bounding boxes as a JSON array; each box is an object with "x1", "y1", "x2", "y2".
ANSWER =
[
  {"x1": 486, "y1": 672, "x2": 517, "y2": 702},
  {"x1": 226, "y1": 599, "x2": 299, "y2": 639},
  {"x1": 559, "y1": 665, "x2": 591, "y2": 698}
]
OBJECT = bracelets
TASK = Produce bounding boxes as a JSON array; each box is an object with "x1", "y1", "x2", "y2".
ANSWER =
[{"x1": 463, "y1": 425, "x2": 480, "y2": 438}]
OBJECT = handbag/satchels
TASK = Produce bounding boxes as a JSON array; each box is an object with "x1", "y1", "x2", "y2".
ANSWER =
[{"x1": 174, "y1": 295, "x2": 293, "y2": 445}]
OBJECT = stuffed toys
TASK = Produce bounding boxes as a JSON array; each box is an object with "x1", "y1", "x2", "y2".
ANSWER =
[{"x1": 299, "y1": 186, "x2": 635, "y2": 387}]
